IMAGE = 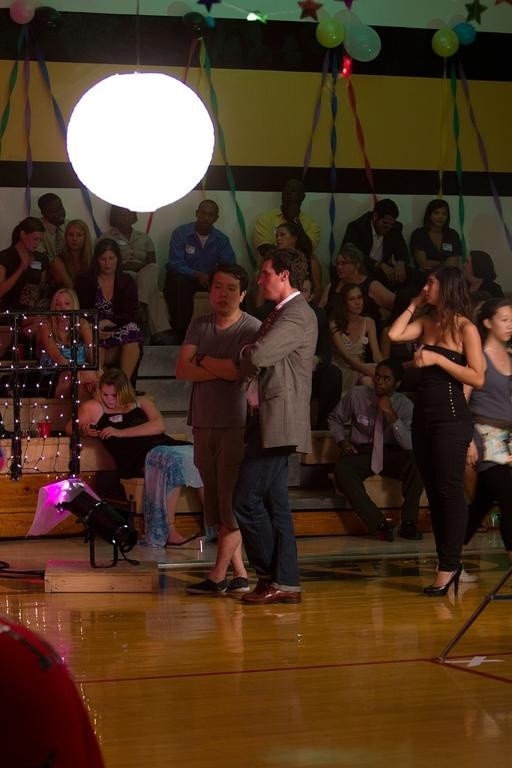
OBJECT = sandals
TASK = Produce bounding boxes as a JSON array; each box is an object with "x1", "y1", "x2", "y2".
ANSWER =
[{"x1": 164, "y1": 521, "x2": 186, "y2": 545}]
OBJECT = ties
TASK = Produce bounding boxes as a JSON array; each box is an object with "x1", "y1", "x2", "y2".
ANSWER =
[
  {"x1": 253, "y1": 308, "x2": 280, "y2": 344},
  {"x1": 369, "y1": 406, "x2": 386, "y2": 476},
  {"x1": 54, "y1": 225, "x2": 66, "y2": 258}
]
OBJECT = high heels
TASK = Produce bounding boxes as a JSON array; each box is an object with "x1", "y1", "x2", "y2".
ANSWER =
[{"x1": 423, "y1": 562, "x2": 463, "y2": 597}]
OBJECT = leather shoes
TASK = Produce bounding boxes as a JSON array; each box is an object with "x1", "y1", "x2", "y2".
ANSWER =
[
  {"x1": 373, "y1": 522, "x2": 396, "y2": 543},
  {"x1": 398, "y1": 523, "x2": 426, "y2": 542},
  {"x1": 243, "y1": 586, "x2": 304, "y2": 606},
  {"x1": 241, "y1": 579, "x2": 273, "y2": 601}
]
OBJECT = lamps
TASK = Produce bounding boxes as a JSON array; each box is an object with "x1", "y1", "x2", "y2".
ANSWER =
[{"x1": 65, "y1": 0, "x2": 214, "y2": 211}]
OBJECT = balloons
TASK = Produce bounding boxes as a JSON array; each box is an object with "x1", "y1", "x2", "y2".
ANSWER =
[
  {"x1": 206, "y1": 17, "x2": 215, "y2": 28},
  {"x1": 345, "y1": 26, "x2": 381, "y2": 61},
  {"x1": 432, "y1": 29, "x2": 459, "y2": 57},
  {"x1": 11, "y1": 1, "x2": 34, "y2": 24},
  {"x1": 316, "y1": 18, "x2": 345, "y2": 48},
  {"x1": 455, "y1": 22, "x2": 477, "y2": 45},
  {"x1": 33, "y1": 6, "x2": 60, "y2": 33}
]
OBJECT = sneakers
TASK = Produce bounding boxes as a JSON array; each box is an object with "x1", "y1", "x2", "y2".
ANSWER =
[
  {"x1": 185, "y1": 578, "x2": 232, "y2": 595},
  {"x1": 225, "y1": 576, "x2": 250, "y2": 592},
  {"x1": 434, "y1": 563, "x2": 477, "y2": 583}
]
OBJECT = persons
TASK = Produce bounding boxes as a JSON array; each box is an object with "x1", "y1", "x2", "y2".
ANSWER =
[
  {"x1": 177, "y1": 252, "x2": 319, "y2": 603},
  {"x1": 65, "y1": 370, "x2": 210, "y2": 546},
  {"x1": 255, "y1": 192, "x2": 502, "y2": 543},
  {"x1": 1, "y1": 616, "x2": 106, "y2": 767},
  {"x1": 387, "y1": 267, "x2": 512, "y2": 598},
  {"x1": 1, "y1": 193, "x2": 172, "y2": 396},
  {"x1": 162, "y1": 198, "x2": 235, "y2": 344}
]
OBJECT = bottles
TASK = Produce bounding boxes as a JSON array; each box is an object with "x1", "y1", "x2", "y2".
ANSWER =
[{"x1": 119, "y1": 238, "x2": 130, "y2": 268}]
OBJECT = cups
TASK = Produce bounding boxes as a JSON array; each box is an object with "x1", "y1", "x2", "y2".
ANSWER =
[
  {"x1": 487, "y1": 511, "x2": 500, "y2": 530},
  {"x1": 37, "y1": 422, "x2": 53, "y2": 437}
]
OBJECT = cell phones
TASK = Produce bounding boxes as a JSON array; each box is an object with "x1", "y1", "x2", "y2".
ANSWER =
[{"x1": 90, "y1": 423, "x2": 98, "y2": 431}]
